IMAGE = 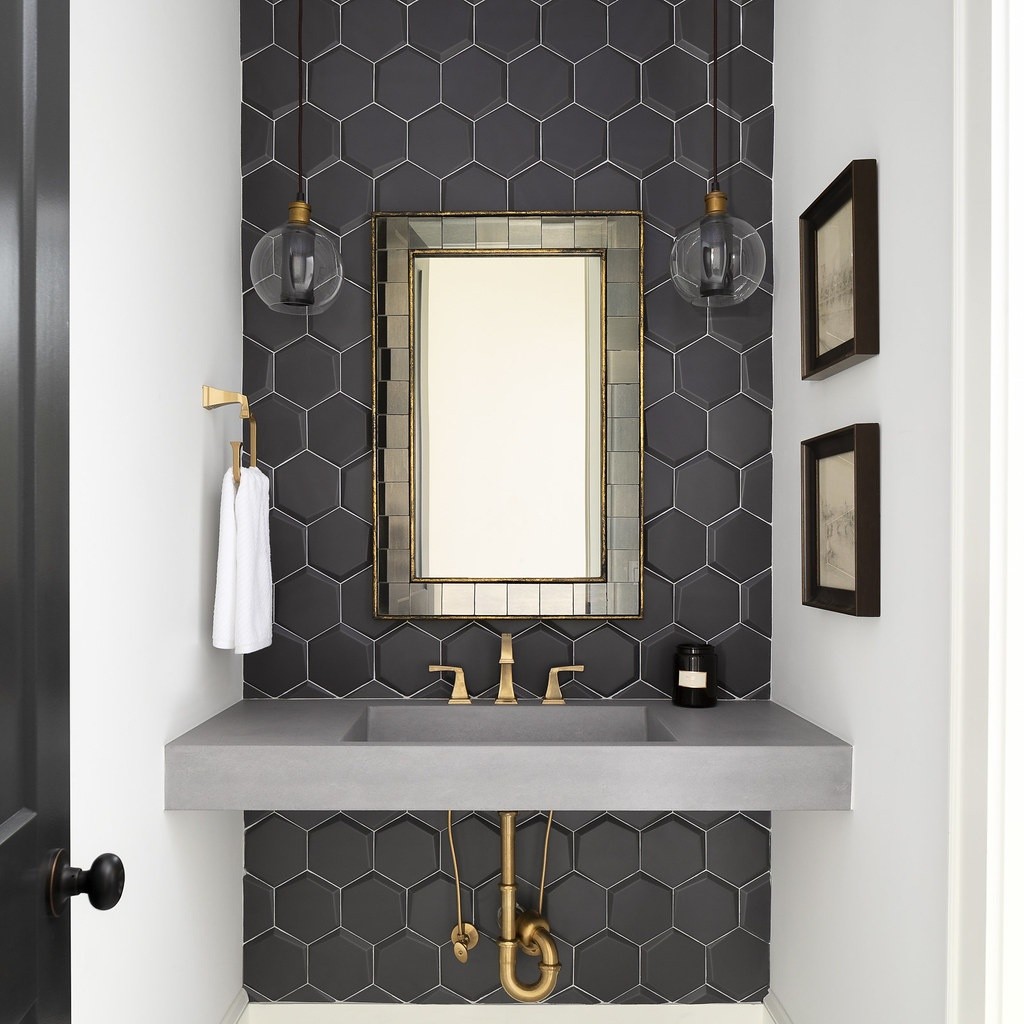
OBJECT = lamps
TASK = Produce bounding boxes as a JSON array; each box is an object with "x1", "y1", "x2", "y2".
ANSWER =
[
  {"x1": 669, "y1": 0, "x2": 767, "y2": 308},
  {"x1": 250, "y1": 0, "x2": 345, "y2": 317}
]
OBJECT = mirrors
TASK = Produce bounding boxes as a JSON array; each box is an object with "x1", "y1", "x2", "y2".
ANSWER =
[{"x1": 408, "y1": 247, "x2": 608, "y2": 584}]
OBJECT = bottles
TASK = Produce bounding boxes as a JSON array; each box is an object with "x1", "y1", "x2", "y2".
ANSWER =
[{"x1": 674, "y1": 644, "x2": 717, "y2": 708}]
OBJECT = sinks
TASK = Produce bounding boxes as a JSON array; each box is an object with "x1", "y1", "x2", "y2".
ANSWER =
[{"x1": 340, "y1": 705, "x2": 678, "y2": 742}]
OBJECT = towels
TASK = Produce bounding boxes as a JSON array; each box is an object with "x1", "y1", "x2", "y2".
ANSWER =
[{"x1": 212, "y1": 466, "x2": 274, "y2": 656}]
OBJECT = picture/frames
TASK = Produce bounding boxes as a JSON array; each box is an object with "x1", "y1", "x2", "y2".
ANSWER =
[
  {"x1": 800, "y1": 423, "x2": 881, "y2": 617},
  {"x1": 797, "y1": 159, "x2": 880, "y2": 382}
]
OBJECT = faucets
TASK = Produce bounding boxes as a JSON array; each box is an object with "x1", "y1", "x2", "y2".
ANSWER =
[{"x1": 493, "y1": 633, "x2": 518, "y2": 705}]
